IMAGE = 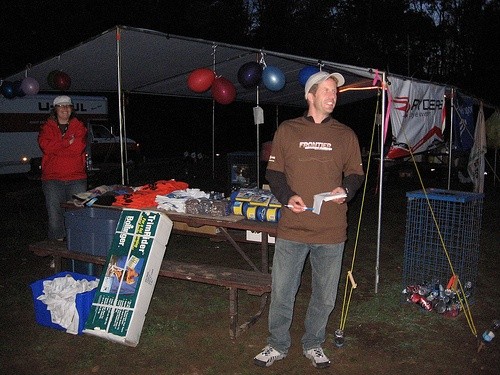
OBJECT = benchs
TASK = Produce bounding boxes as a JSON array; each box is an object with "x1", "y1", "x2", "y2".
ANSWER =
[
  {"x1": 27, "y1": 240, "x2": 272, "y2": 341},
  {"x1": 171, "y1": 229, "x2": 275, "y2": 273}
]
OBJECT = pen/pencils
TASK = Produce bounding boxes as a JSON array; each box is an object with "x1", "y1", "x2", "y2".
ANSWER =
[{"x1": 284, "y1": 205, "x2": 314, "y2": 210}]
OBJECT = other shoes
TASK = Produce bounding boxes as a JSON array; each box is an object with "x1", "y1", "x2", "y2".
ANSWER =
[{"x1": 34, "y1": 238, "x2": 67, "y2": 256}]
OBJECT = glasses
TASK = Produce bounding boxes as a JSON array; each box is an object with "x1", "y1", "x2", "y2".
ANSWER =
[{"x1": 56, "y1": 104, "x2": 72, "y2": 108}]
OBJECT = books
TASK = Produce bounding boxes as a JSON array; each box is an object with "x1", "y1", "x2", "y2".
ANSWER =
[{"x1": 312, "y1": 192, "x2": 348, "y2": 216}]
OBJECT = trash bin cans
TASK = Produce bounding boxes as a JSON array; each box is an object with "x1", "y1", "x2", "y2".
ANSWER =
[
  {"x1": 64, "y1": 208, "x2": 123, "y2": 276},
  {"x1": 29, "y1": 271, "x2": 100, "y2": 336},
  {"x1": 227, "y1": 151, "x2": 258, "y2": 196},
  {"x1": 401, "y1": 188, "x2": 485, "y2": 318}
]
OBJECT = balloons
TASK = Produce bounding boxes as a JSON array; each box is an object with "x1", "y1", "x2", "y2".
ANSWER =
[
  {"x1": 1, "y1": 80, "x2": 16, "y2": 99},
  {"x1": 21, "y1": 77, "x2": 39, "y2": 95},
  {"x1": 298, "y1": 65, "x2": 319, "y2": 86},
  {"x1": 14, "y1": 79, "x2": 26, "y2": 97},
  {"x1": 261, "y1": 64, "x2": 286, "y2": 91},
  {"x1": 212, "y1": 77, "x2": 236, "y2": 104},
  {"x1": 238, "y1": 61, "x2": 263, "y2": 88},
  {"x1": 54, "y1": 72, "x2": 71, "y2": 90},
  {"x1": 188, "y1": 68, "x2": 215, "y2": 93}
]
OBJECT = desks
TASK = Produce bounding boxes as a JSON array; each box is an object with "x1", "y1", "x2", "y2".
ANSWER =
[{"x1": 59, "y1": 198, "x2": 277, "y2": 299}]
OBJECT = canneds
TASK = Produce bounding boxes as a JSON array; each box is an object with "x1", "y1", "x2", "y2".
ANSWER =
[
  {"x1": 406, "y1": 281, "x2": 475, "y2": 318},
  {"x1": 335, "y1": 329, "x2": 344, "y2": 347}
]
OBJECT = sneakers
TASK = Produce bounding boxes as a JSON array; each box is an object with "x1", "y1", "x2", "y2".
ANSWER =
[
  {"x1": 254, "y1": 344, "x2": 286, "y2": 366},
  {"x1": 303, "y1": 346, "x2": 331, "y2": 368}
]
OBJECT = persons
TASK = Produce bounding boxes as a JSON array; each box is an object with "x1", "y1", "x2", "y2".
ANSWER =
[
  {"x1": 36, "y1": 95, "x2": 89, "y2": 247},
  {"x1": 251, "y1": 71, "x2": 365, "y2": 369}
]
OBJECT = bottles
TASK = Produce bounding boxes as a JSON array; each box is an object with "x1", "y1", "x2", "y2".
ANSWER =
[
  {"x1": 335, "y1": 330, "x2": 344, "y2": 348},
  {"x1": 407, "y1": 275, "x2": 471, "y2": 317},
  {"x1": 481, "y1": 319, "x2": 500, "y2": 345}
]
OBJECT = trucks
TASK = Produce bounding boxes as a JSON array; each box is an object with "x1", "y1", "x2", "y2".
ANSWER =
[{"x1": 0, "y1": 92, "x2": 140, "y2": 181}]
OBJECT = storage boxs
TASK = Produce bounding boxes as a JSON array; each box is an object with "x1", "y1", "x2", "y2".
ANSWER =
[
  {"x1": 63, "y1": 208, "x2": 121, "y2": 257},
  {"x1": 28, "y1": 271, "x2": 101, "y2": 336}
]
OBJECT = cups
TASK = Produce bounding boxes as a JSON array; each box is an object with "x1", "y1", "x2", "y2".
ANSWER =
[{"x1": 185, "y1": 191, "x2": 232, "y2": 216}]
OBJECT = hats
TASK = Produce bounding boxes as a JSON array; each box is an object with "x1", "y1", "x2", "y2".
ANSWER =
[
  {"x1": 305, "y1": 70, "x2": 345, "y2": 99},
  {"x1": 54, "y1": 95, "x2": 72, "y2": 106}
]
